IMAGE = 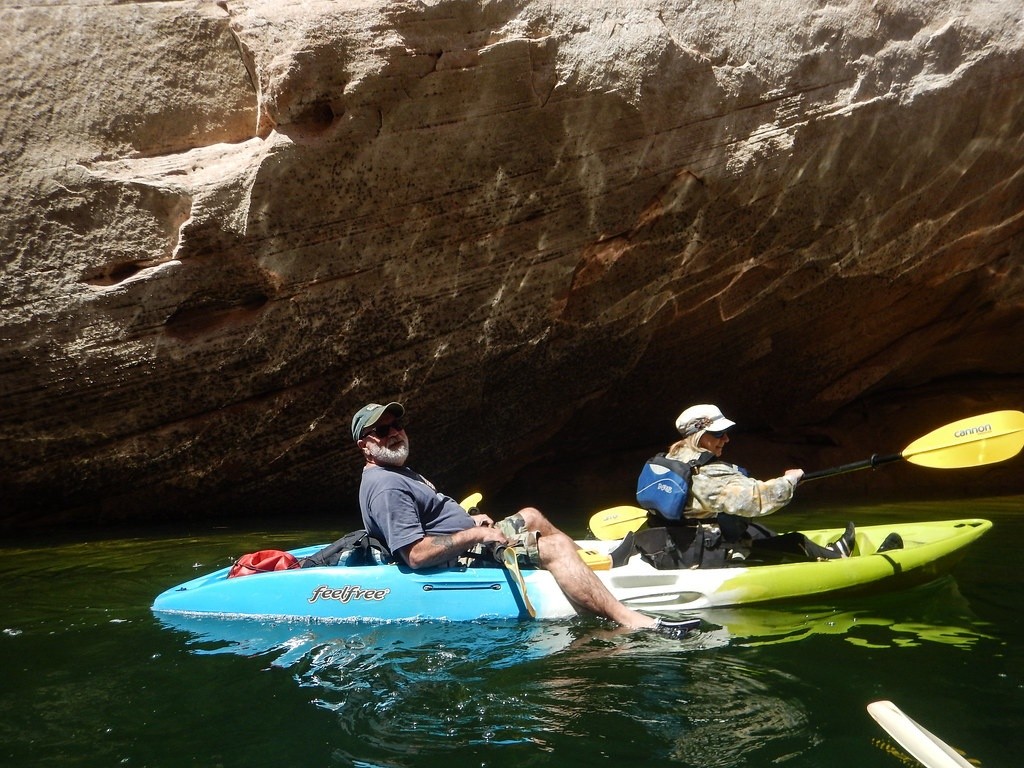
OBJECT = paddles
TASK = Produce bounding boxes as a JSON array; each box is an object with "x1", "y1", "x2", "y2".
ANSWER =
[
  {"x1": 868, "y1": 700, "x2": 973, "y2": 768},
  {"x1": 589, "y1": 408, "x2": 1024, "y2": 540},
  {"x1": 456, "y1": 490, "x2": 539, "y2": 623}
]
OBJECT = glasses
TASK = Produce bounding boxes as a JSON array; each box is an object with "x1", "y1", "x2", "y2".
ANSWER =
[
  {"x1": 707, "y1": 429, "x2": 728, "y2": 438},
  {"x1": 361, "y1": 419, "x2": 403, "y2": 439}
]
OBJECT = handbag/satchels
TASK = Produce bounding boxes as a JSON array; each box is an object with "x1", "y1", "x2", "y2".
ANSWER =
[
  {"x1": 227, "y1": 550, "x2": 300, "y2": 578},
  {"x1": 302, "y1": 531, "x2": 392, "y2": 567}
]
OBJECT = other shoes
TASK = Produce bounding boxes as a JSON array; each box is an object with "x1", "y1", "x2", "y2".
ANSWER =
[
  {"x1": 876, "y1": 533, "x2": 903, "y2": 553},
  {"x1": 825, "y1": 521, "x2": 856, "y2": 557},
  {"x1": 654, "y1": 614, "x2": 702, "y2": 640},
  {"x1": 610, "y1": 531, "x2": 634, "y2": 568}
]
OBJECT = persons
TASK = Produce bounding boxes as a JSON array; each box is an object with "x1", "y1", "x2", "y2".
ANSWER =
[
  {"x1": 634, "y1": 404, "x2": 904, "y2": 570},
  {"x1": 351, "y1": 402, "x2": 701, "y2": 632}
]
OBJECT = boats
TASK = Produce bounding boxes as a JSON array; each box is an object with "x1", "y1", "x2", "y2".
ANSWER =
[{"x1": 153, "y1": 518, "x2": 993, "y2": 620}]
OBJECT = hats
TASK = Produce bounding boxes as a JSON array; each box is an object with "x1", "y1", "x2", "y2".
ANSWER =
[
  {"x1": 675, "y1": 404, "x2": 736, "y2": 437},
  {"x1": 351, "y1": 402, "x2": 405, "y2": 445}
]
